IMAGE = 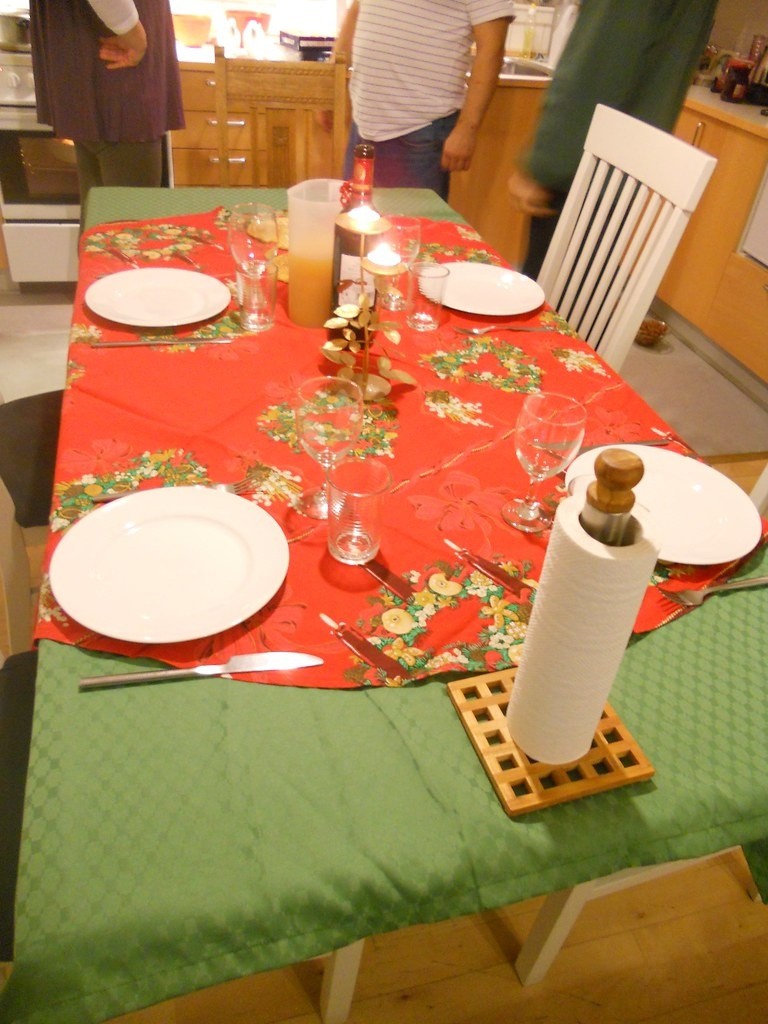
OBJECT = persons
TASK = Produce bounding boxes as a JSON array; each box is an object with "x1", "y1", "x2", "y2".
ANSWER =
[
  {"x1": 505, "y1": 0, "x2": 720, "y2": 282},
  {"x1": 28, "y1": 0, "x2": 188, "y2": 249},
  {"x1": 332, "y1": 0, "x2": 519, "y2": 199}
]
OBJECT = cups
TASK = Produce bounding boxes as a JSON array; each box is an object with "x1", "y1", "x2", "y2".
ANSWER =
[
  {"x1": 326, "y1": 458, "x2": 392, "y2": 566},
  {"x1": 405, "y1": 263, "x2": 450, "y2": 332},
  {"x1": 235, "y1": 260, "x2": 278, "y2": 332}
]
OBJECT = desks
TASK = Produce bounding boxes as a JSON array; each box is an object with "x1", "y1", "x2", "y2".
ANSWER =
[{"x1": 0, "y1": 187, "x2": 768, "y2": 1024}]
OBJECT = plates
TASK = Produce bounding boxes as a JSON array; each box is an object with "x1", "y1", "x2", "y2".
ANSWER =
[
  {"x1": 564, "y1": 443, "x2": 762, "y2": 566},
  {"x1": 84, "y1": 267, "x2": 231, "y2": 329},
  {"x1": 47, "y1": 487, "x2": 288, "y2": 645},
  {"x1": 418, "y1": 261, "x2": 546, "y2": 317}
]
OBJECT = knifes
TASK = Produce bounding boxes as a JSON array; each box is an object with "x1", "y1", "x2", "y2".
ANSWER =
[
  {"x1": 90, "y1": 338, "x2": 234, "y2": 348},
  {"x1": 78, "y1": 652, "x2": 324, "y2": 692},
  {"x1": 528, "y1": 439, "x2": 670, "y2": 457}
]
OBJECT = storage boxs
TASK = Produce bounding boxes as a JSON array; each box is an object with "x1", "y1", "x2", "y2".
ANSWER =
[{"x1": 278, "y1": 30, "x2": 336, "y2": 49}]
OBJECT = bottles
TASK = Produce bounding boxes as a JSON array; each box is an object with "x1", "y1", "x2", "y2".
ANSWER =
[{"x1": 330, "y1": 145, "x2": 383, "y2": 352}]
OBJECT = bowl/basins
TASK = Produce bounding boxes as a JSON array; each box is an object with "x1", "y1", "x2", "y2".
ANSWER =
[{"x1": 635, "y1": 320, "x2": 671, "y2": 347}]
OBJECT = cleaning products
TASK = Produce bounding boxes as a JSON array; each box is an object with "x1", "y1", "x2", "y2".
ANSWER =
[{"x1": 520, "y1": 2, "x2": 540, "y2": 58}]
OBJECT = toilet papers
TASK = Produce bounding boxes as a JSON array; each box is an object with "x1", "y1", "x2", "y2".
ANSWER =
[{"x1": 507, "y1": 493, "x2": 665, "y2": 763}]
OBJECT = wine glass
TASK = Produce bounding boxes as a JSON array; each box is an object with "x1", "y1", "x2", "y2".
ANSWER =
[
  {"x1": 295, "y1": 376, "x2": 363, "y2": 520},
  {"x1": 375, "y1": 214, "x2": 421, "y2": 313},
  {"x1": 227, "y1": 202, "x2": 279, "y2": 309},
  {"x1": 502, "y1": 393, "x2": 586, "y2": 533}
]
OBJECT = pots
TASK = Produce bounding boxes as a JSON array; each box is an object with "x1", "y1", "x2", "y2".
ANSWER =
[{"x1": 0, "y1": 9, "x2": 30, "y2": 53}]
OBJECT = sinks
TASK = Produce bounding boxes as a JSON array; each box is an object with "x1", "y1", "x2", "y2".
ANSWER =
[{"x1": 464, "y1": 53, "x2": 550, "y2": 82}]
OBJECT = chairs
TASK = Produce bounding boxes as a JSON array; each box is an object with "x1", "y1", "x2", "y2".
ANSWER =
[{"x1": 0, "y1": 43, "x2": 768, "y2": 1024}]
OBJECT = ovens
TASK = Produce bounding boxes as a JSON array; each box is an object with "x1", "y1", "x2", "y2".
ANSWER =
[{"x1": 1, "y1": 105, "x2": 174, "y2": 287}]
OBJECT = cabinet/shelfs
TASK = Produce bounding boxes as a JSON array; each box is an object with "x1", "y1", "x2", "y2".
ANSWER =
[
  {"x1": 701, "y1": 165, "x2": 768, "y2": 384},
  {"x1": 445, "y1": 87, "x2": 551, "y2": 264},
  {"x1": 172, "y1": 70, "x2": 347, "y2": 187},
  {"x1": 649, "y1": 103, "x2": 768, "y2": 327}
]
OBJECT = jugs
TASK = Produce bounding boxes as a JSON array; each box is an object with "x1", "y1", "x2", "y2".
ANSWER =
[{"x1": 286, "y1": 178, "x2": 347, "y2": 326}]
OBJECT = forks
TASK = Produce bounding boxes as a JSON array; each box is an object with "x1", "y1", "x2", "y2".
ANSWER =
[
  {"x1": 90, "y1": 471, "x2": 267, "y2": 504},
  {"x1": 655, "y1": 576, "x2": 767, "y2": 607},
  {"x1": 451, "y1": 325, "x2": 554, "y2": 338}
]
